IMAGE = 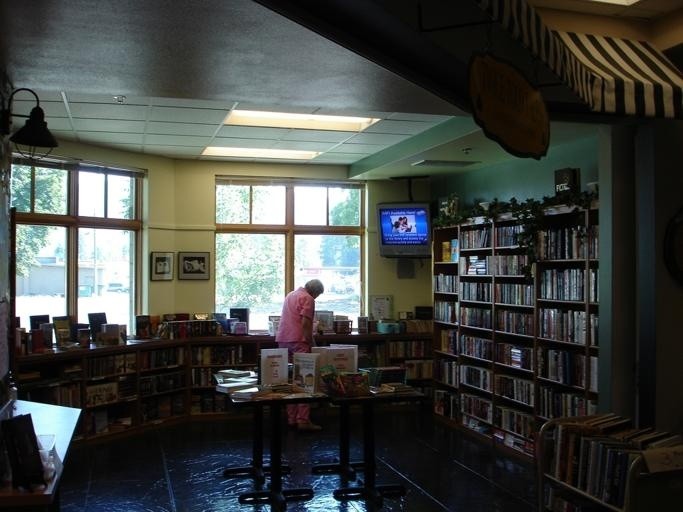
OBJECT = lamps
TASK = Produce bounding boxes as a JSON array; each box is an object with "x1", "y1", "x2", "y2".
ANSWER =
[{"x1": 0, "y1": 88, "x2": 59, "y2": 160}]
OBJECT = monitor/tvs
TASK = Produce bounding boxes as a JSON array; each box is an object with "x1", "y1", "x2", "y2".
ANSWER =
[{"x1": 376, "y1": 201, "x2": 431, "y2": 259}]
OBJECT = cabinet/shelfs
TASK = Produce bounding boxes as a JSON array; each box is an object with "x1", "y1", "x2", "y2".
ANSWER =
[
  {"x1": 18, "y1": 331, "x2": 430, "y2": 450},
  {"x1": 431, "y1": 201, "x2": 683, "y2": 512}
]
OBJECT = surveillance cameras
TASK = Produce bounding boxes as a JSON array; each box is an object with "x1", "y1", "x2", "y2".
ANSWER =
[{"x1": 462, "y1": 147, "x2": 472, "y2": 154}]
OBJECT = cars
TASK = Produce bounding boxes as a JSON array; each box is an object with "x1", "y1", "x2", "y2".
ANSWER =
[
  {"x1": 297, "y1": 272, "x2": 360, "y2": 296},
  {"x1": 106, "y1": 282, "x2": 125, "y2": 292}
]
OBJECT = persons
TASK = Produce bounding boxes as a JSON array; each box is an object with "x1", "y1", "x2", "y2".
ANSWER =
[
  {"x1": 392, "y1": 217, "x2": 408, "y2": 233},
  {"x1": 275, "y1": 279, "x2": 324, "y2": 430}
]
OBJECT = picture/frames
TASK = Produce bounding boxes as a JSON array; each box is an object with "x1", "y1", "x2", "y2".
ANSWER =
[
  {"x1": 178, "y1": 251, "x2": 210, "y2": 279},
  {"x1": 152, "y1": 252, "x2": 173, "y2": 281}
]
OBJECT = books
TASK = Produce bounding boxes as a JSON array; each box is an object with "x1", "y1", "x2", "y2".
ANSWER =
[
  {"x1": 15, "y1": 308, "x2": 260, "y2": 442},
  {"x1": 432, "y1": 224, "x2": 600, "y2": 457},
  {"x1": 548, "y1": 413, "x2": 683, "y2": 511},
  {"x1": 330, "y1": 319, "x2": 432, "y2": 405},
  {"x1": 260, "y1": 348, "x2": 289, "y2": 386},
  {"x1": 293, "y1": 352, "x2": 320, "y2": 394}
]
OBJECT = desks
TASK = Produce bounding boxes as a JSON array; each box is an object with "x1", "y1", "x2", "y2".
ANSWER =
[
  {"x1": 218, "y1": 380, "x2": 426, "y2": 512},
  {"x1": 0, "y1": 399, "x2": 83, "y2": 512}
]
